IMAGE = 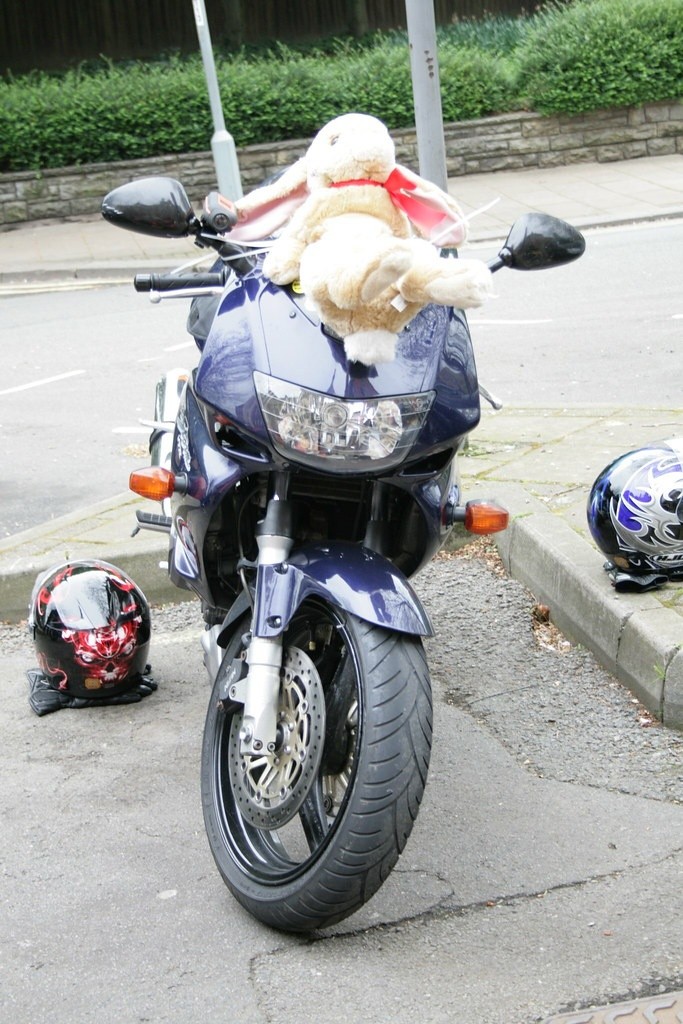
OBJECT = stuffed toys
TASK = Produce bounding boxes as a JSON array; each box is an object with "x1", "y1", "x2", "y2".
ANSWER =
[{"x1": 223, "y1": 112, "x2": 495, "y2": 367}]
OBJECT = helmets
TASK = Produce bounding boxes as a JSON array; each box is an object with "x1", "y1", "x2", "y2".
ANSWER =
[
  {"x1": 30, "y1": 559, "x2": 151, "y2": 697},
  {"x1": 587, "y1": 446, "x2": 683, "y2": 573}
]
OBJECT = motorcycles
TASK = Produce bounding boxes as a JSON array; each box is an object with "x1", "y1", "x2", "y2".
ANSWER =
[{"x1": 100, "y1": 176, "x2": 587, "y2": 937}]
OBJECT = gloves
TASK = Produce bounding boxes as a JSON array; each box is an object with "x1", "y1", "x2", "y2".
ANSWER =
[
  {"x1": 25, "y1": 663, "x2": 159, "y2": 718},
  {"x1": 603, "y1": 562, "x2": 669, "y2": 593}
]
